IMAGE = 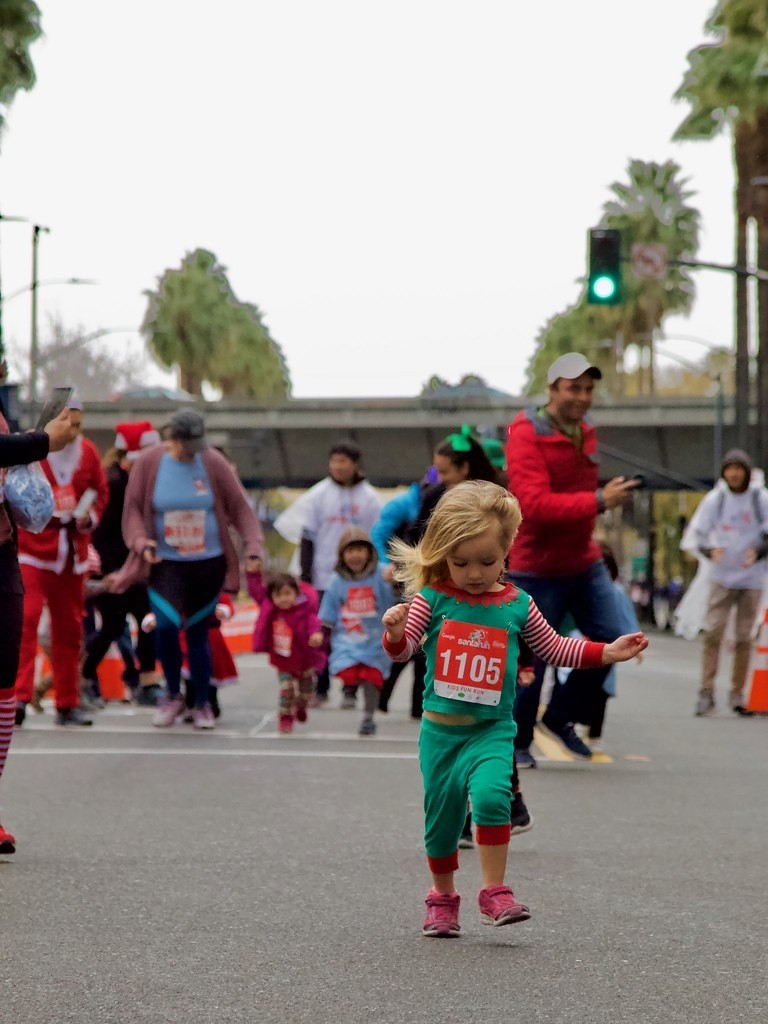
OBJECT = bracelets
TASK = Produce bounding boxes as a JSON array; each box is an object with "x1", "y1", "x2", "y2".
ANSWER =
[{"x1": 595, "y1": 488, "x2": 605, "y2": 515}]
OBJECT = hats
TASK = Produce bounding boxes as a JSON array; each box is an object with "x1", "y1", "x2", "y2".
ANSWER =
[
  {"x1": 547, "y1": 353, "x2": 602, "y2": 384},
  {"x1": 723, "y1": 448, "x2": 751, "y2": 471},
  {"x1": 170, "y1": 410, "x2": 208, "y2": 453},
  {"x1": 114, "y1": 422, "x2": 164, "y2": 460}
]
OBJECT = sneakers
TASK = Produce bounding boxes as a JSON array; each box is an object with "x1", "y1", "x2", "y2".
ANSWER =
[
  {"x1": 539, "y1": 715, "x2": 591, "y2": 759},
  {"x1": 479, "y1": 884, "x2": 530, "y2": 926},
  {"x1": 152, "y1": 692, "x2": 186, "y2": 727},
  {"x1": 190, "y1": 703, "x2": 215, "y2": 729},
  {"x1": 458, "y1": 812, "x2": 474, "y2": 847},
  {"x1": 514, "y1": 750, "x2": 536, "y2": 768},
  {"x1": 423, "y1": 891, "x2": 462, "y2": 935},
  {"x1": 510, "y1": 792, "x2": 531, "y2": 834}
]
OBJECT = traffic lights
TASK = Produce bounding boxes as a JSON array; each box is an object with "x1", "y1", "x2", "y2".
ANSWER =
[{"x1": 587, "y1": 228, "x2": 621, "y2": 305}]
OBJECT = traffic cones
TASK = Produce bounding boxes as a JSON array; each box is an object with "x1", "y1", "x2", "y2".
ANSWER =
[
  {"x1": 97, "y1": 602, "x2": 260, "y2": 703},
  {"x1": 746, "y1": 608, "x2": 767, "y2": 714}
]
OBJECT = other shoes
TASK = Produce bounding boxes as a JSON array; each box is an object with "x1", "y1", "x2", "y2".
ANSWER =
[
  {"x1": 14, "y1": 702, "x2": 25, "y2": 726},
  {"x1": 28, "y1": 685, "x2": 43, "y2": 712},
  {"x1": 279, "y1": 712, "x2": 293, "y2": 732},
  {"x1": 697, "y1": 694, "x2": 715, "y2": 717},
  {"x1": 360, "y1": 720, "x2": 375, "y2": 734},
  {"x1": 0, "y1": 825, "x2": 17, "y2": 852},
  {"x1": 54, "y1": 709, "x2": 92, "y2": 726},
  {"x1": 310, "y1": 692, "x2": 326, "y2": 708},
  {"x1": 730, "y1": 692, "x2": 744, "y2": 712},
  {"x1": 344, "y1": 695, "x2": 356, "y2": 708}
]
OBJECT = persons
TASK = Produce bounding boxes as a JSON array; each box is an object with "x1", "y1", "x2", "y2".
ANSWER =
[
  {"x1": 316, "y1": 528, "x2": 397, "y2": 736},
  {"x1": 247, "y1": 566, "x2": 327, "y2": 736},
  {"x1": 140, "y1": 592, "x2": 239, "y2": 723},
  {"x1": 677, "y1": 447, "x2": 768, "y2": 718},
  {"x1": 29, "y1": 420, "x2": 161, "y2": 714},
  {"x1": 123, "y1": 411, "x2": 264, "y2": 728},
  {"x1": 505, "y1": 351, "x2": 640, "y2": 768},
  {"x1": 0, "y1": 407, "x2": 72, "y2": 855},
  {"x1": 15, "y1": 399, "x2": 109, "y2": 727},
  {"x1": 370, "y1": 423, "x2": 510, "y2": 723},
  {"x1": 273, "y1": 442, "x2": 383, "y2": 710},
  {"x1": 382, "y1": 479, "x2": 650, "y2": 936}
]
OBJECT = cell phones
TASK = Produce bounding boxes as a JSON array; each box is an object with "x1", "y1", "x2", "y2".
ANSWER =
[{"x1": 35, "y1": 386, "x2": 74, "y2": 432}]
